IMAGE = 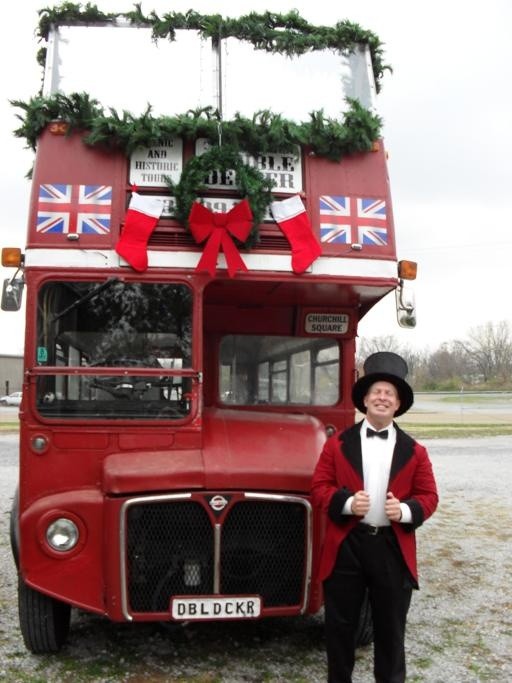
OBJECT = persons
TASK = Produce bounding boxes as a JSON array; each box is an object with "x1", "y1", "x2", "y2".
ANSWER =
[{"x1": 311, "y1": 381, "x2": 439, "y2": 683}]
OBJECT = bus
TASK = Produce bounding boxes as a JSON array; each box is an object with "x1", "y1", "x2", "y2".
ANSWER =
[{"x1": 1, "y1": 9, "x2": 416, "y2": 654}]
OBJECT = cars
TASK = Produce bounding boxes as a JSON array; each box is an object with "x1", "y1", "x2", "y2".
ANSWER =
[{"x1": 0, "y1": 392, "x2": 23, "y2": 407}]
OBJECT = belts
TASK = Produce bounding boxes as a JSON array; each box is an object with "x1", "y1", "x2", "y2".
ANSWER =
[{"x1": 362, "y1": 526, "x2": 392, "y2": 536}]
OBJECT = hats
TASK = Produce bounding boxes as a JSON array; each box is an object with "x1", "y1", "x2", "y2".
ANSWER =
[{"x1": 351, "y1": 351, "x2": 414, "y2": 417}]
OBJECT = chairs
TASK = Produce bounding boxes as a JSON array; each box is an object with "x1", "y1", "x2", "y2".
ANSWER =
[{"x1": 90, "y1": 371, "x2": 159, "y2": 398}]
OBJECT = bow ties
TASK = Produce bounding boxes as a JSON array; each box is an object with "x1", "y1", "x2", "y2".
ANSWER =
[{"x1": 367, "y1": 428, "x2": 388, "y2": 439}]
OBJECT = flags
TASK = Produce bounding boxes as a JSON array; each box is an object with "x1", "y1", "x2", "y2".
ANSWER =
[
  {"x1": 319, "y1": 194, "x2": 387, "y2": 246},
  {"x1": 36, "y1": 183, "x2": 113, "y2": 234}
]
